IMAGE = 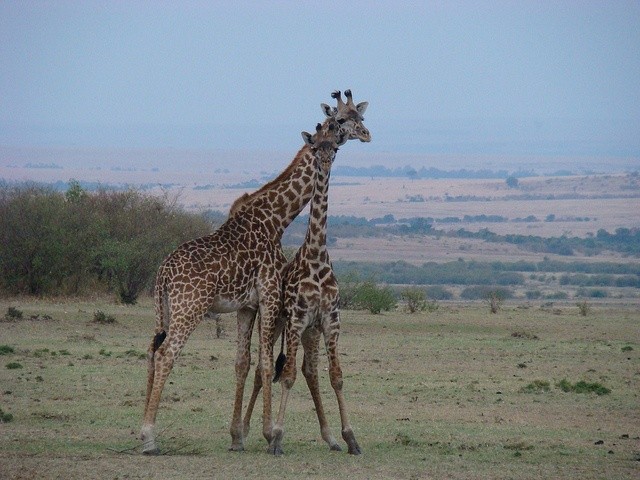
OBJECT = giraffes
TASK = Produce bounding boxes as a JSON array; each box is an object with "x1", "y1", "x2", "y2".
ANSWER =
[
  {"x1": 244, "y1": 123, "x2": 361, "y2": 455},
  {"x1": 139, "y1": 89, "x2": 372, "y2": 455}
]
